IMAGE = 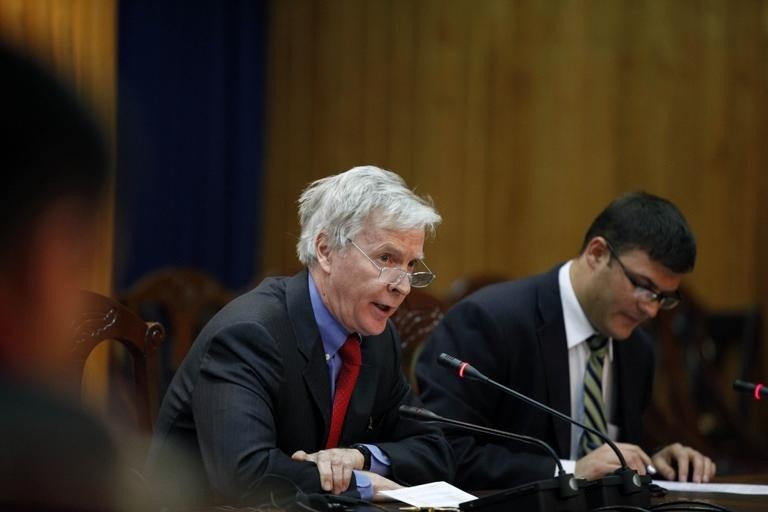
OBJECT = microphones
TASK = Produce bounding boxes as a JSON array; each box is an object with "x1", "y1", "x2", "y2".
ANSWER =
[
  {"x1": 732, "y1": 380, "x2": 768, "y2": 401},
  {"x1": 397, "y1": 404, "x2": 602, "y2": 512},
  {"x1": 437, "y1": 351, "x2": 653, "y2": 512}
]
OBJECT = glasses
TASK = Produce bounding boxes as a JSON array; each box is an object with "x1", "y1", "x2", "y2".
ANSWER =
[
  {"x1": 599, "y1": 238, "x2": 681, "y2": 312},
  {"x1": 340, "y1": 229, "x2": 438, "y2": 289}
]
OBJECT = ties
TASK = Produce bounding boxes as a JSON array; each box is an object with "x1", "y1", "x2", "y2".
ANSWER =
[
  {"x1": 324, "y1": 331, "x2": 364, "y2": 456},
  {"x1": 577, "y1": 330, "x2": 611, "y2": 463}
]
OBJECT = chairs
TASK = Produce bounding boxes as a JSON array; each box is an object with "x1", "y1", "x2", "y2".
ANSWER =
[{"x1": 0, "y1": 269, "x2": 515, "y2": 473}]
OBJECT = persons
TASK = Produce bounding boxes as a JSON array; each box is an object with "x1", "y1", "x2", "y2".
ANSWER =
[
  {"x1": 410, "y1": 188, "x2": 722, "y2": 490},
  {"x1": 132, "y1": 159, "x2": 459, "y2": 512},
  {"x1": 0, "y1": 25, "x2": 199, "y2": 512}
]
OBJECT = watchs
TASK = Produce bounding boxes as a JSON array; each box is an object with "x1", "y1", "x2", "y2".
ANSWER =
[{"x1": 352, "y1": 445, "x2": 371, "y2": 473}]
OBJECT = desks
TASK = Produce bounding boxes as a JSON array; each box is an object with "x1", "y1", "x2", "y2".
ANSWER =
[{"x1": 344, "y1": 475, "x2": 768, "y2": 512}]
